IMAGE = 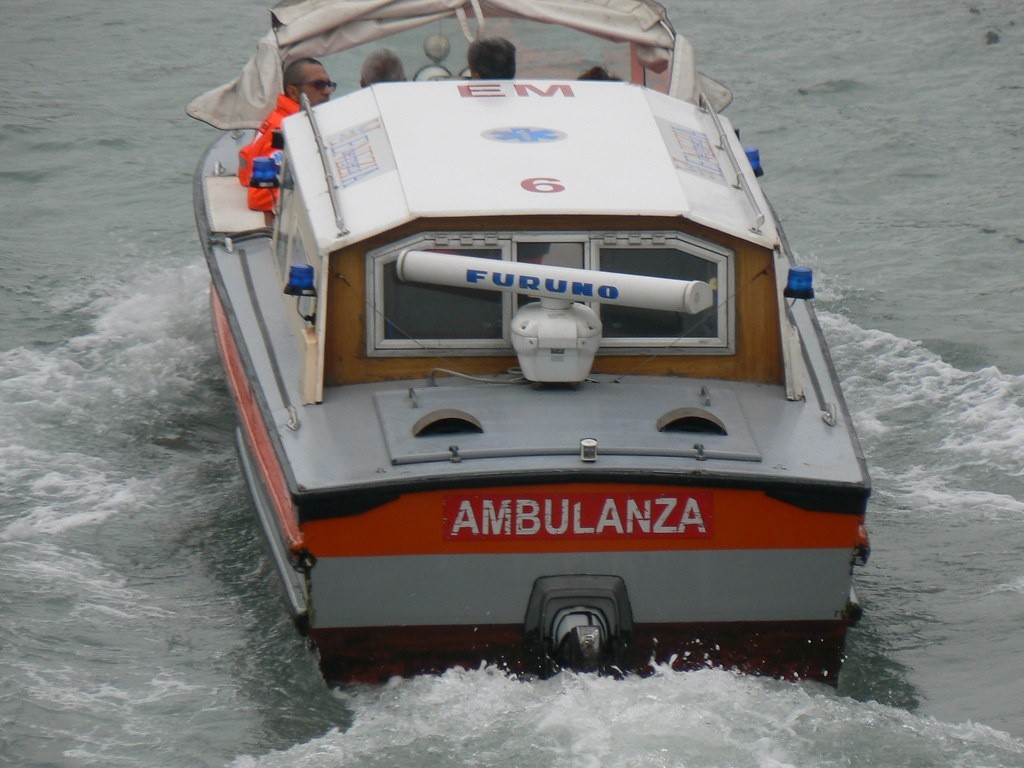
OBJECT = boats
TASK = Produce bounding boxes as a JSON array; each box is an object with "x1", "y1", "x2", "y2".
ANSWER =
[{"x1": 185, "y1": 1, "x2": 876, "y2": 693}]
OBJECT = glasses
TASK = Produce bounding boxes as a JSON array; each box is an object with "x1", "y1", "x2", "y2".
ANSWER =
[{"x1": 290, "y1": 80, "x2": 338, "y2": 92}]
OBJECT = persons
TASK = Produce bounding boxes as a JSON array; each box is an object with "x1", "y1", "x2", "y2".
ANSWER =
[
  {"x1": 236, "y1": 55, "x2": 334, "y2": 229},
  {"x1": 360, "y1": 49, "x2": 408, "y2": 88},
  {"x1": 468, "y1": 35, "x2": 515, "y2": 81}
]
